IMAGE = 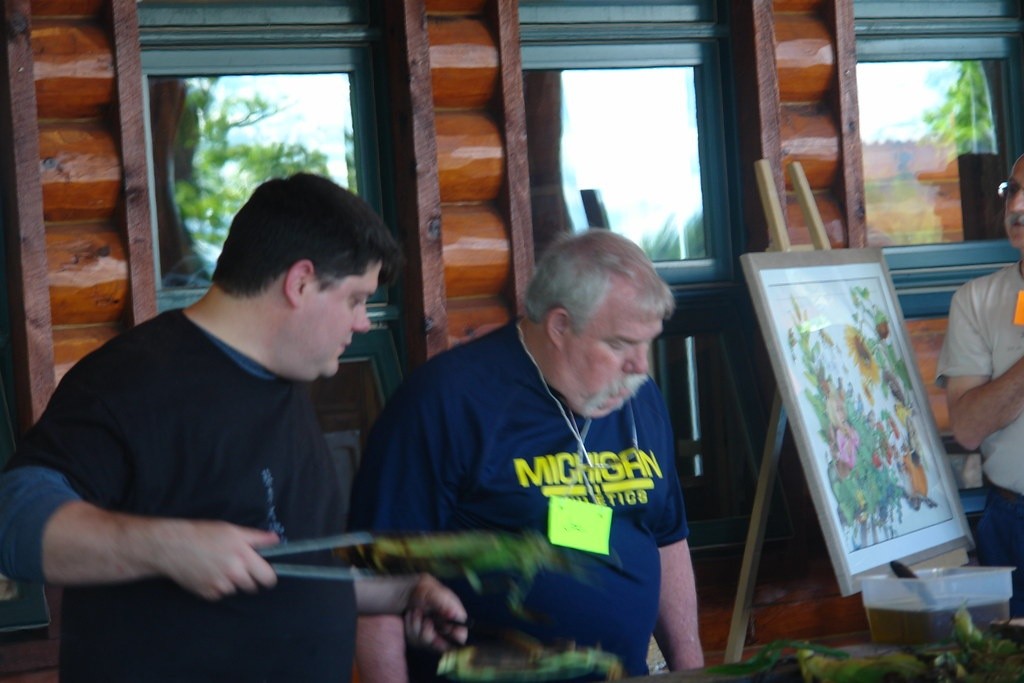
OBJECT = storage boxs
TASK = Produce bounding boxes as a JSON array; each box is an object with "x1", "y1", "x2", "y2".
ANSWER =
[{"x1": 861, "y1": 563, "x2": 1017, "y2": 647}]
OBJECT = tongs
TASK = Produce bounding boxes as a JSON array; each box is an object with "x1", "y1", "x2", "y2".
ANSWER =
[{"x1": 255, "y1": 531, "x2": 380, "y2": 582}]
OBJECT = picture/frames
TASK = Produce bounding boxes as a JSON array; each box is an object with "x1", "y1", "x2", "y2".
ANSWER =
[{"x1": 739, "y1": 244, "x2": 977, "y2": 598}]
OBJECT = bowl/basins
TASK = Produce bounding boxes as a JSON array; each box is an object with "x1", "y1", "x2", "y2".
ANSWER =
[{"x1": 857, "y1": 565, "x2": 1018, "y2": 645}]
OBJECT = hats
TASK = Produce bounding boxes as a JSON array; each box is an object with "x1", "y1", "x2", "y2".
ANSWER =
[{"x1": 918, "y1": 158, "x2": 960, "y2": 185}]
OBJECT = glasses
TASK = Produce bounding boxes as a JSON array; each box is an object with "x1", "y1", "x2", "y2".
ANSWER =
[{"x1": 996, "y1": 181, "x2": 1024, "y2": 198}]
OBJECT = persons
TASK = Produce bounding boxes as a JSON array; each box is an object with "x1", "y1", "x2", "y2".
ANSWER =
[
  {"x1": 911, "y1": 156, "x2": 1017, "y2": 243},
  {"x1": 343, "y1": 223, "x2": 705, "y2": 683},
  {"x1": 0, "y1": 169, "x2": 469, "y2": 681},
  {"x1": 928, "y1": 148, "x2": 1024, "y2": 627}
]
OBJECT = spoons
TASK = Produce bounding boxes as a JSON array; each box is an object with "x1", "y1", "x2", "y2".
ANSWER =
[{"x1": 891, "y1": 561, "x2": 938, "y2": 607}]
